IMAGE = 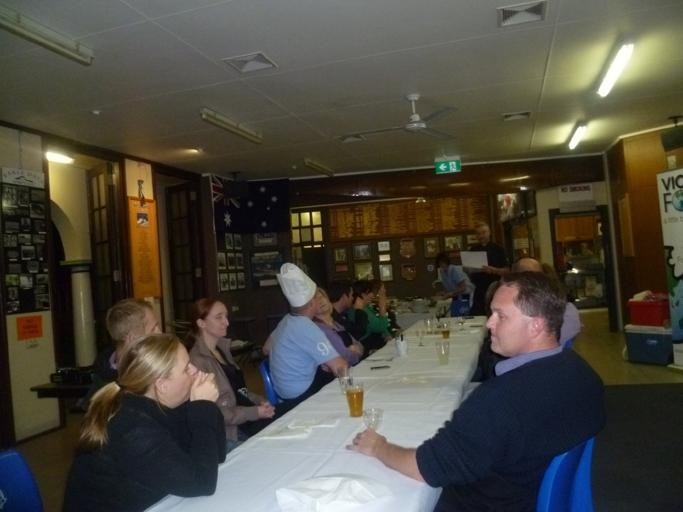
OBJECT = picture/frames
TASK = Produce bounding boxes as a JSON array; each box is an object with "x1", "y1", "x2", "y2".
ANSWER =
[{"x1": 334, "y1": 233, "x2": 481, "y2": 282}]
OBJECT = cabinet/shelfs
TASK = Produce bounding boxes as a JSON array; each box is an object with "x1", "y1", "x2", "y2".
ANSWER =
[{"x1": 164, "y1": 173, "x2": 257, "y2": 335}]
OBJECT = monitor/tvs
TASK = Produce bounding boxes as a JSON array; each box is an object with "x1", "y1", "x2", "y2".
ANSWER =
[{"x1": 497, "y1": 189, "x2": 537, "y2": 221}]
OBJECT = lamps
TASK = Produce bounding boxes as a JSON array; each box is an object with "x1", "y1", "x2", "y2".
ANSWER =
[
  {"x1": 2, "y1": 7, "x2": 94, "y2": 66},
  {"x1": 568, "y1": 122, "x2": 590, "y2": 151},
  {"x1": 593, "y1": 39, "x2": 635, "y2": 97},
  {"x1": 200, "y1": 107, "x2": 264, "y2": 144},
  {"x1": 302, "y1": 158, "x2": 335, "y2": 179}
]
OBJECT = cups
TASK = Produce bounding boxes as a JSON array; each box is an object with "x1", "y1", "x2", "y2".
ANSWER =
[
  {"x1": 338, "y1": 366, "x2": 353, "y2": 392},
  {"x1": 395, "y1": 312, "x2": 466, "y2": 364},
  {"x1": 344, "y1": 383, "x2": 365, "y2": 418}
]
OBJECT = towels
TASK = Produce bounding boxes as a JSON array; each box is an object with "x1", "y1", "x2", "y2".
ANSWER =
[
  {"x1": 276, "y1": 476, "x2": 379, "y2": 512},
  {"x1": 257, "y1": 416, "x2": 343, "y2": 439}
]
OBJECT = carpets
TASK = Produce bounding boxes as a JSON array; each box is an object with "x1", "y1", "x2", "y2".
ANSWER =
[{"x1": 599, "y1": 383, "x2": 683, "y2": 512}]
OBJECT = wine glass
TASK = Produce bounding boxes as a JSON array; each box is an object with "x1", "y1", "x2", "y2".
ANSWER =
[{"x1": 363, "y1": 408, "x2": 383, "y2": 431}]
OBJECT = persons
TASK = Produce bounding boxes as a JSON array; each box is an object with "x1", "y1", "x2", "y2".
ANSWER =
[
  {"x1": 189, "y1": 298, "x2": 276, "y2": 441},
  {"x1": 317, "y1": 277, "x2": 403, "y2": 374},
  {"x1": 432, "y1": 224, "x2": 584, "y2": 395},
  {"x1": 79, "y1": 297, "x2": 163, "y2": 412},
  {"x1": 261, "y1": 262, "x2": 350, "y2": 408},
  {"x1": 63, "y1": 336, "x2": 226, "y2": 512},
  {"x1": 344, "y1": 271, "x2": 608, "y2": 512}
]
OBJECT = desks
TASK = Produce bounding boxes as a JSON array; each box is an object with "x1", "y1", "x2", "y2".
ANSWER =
[{"x1": 29, "y1": 343, "x2": 254, "y2": 428}]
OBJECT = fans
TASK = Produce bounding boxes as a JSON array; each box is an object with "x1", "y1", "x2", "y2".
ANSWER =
[{"x1": 355, "y1": 93, "x2": 460, "y2": 142}]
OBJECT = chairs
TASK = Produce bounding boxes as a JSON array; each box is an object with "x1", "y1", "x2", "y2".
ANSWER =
[
  {"x1": 1, "y1": 453, "x2": 43, "y2": 511},
  {"x1": 257, "y1": 359, "x2": 279, "y2": 407},
  {"x1": 535, "y1": 436, "x2": 602, "y2": 512}
]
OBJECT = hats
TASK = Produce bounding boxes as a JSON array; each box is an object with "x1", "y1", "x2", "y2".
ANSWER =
[{"x1": 277, "y1": 262, "x2": 317, "y2": 307}]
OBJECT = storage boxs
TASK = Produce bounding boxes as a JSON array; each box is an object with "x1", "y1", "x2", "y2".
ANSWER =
[
  {"x1": 625, "y1": 325, "x2": 673, "y2": 367},
  {"x1": 629, "y1": 299, "x2": 669, "y2": 326}
]
